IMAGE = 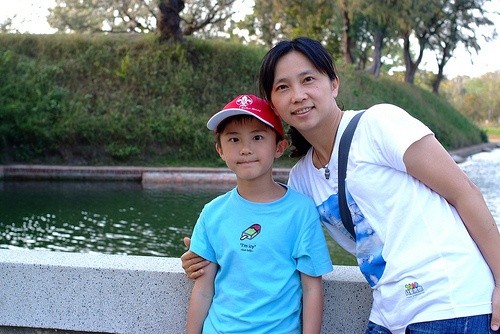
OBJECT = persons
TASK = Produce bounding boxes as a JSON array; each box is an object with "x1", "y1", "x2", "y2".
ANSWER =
[{"x1": 182, "y1": 36, "x2": 499, "y2": 333}]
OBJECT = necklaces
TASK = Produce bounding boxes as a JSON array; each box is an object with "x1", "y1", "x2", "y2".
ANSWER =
[{"x1": 313, "y1": 112, "x2": 343, "y2": 179}]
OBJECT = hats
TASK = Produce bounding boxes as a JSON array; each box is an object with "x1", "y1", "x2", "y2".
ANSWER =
[{"x1": 206, "y1": 94, "x2": 285, "y2": 141}]
